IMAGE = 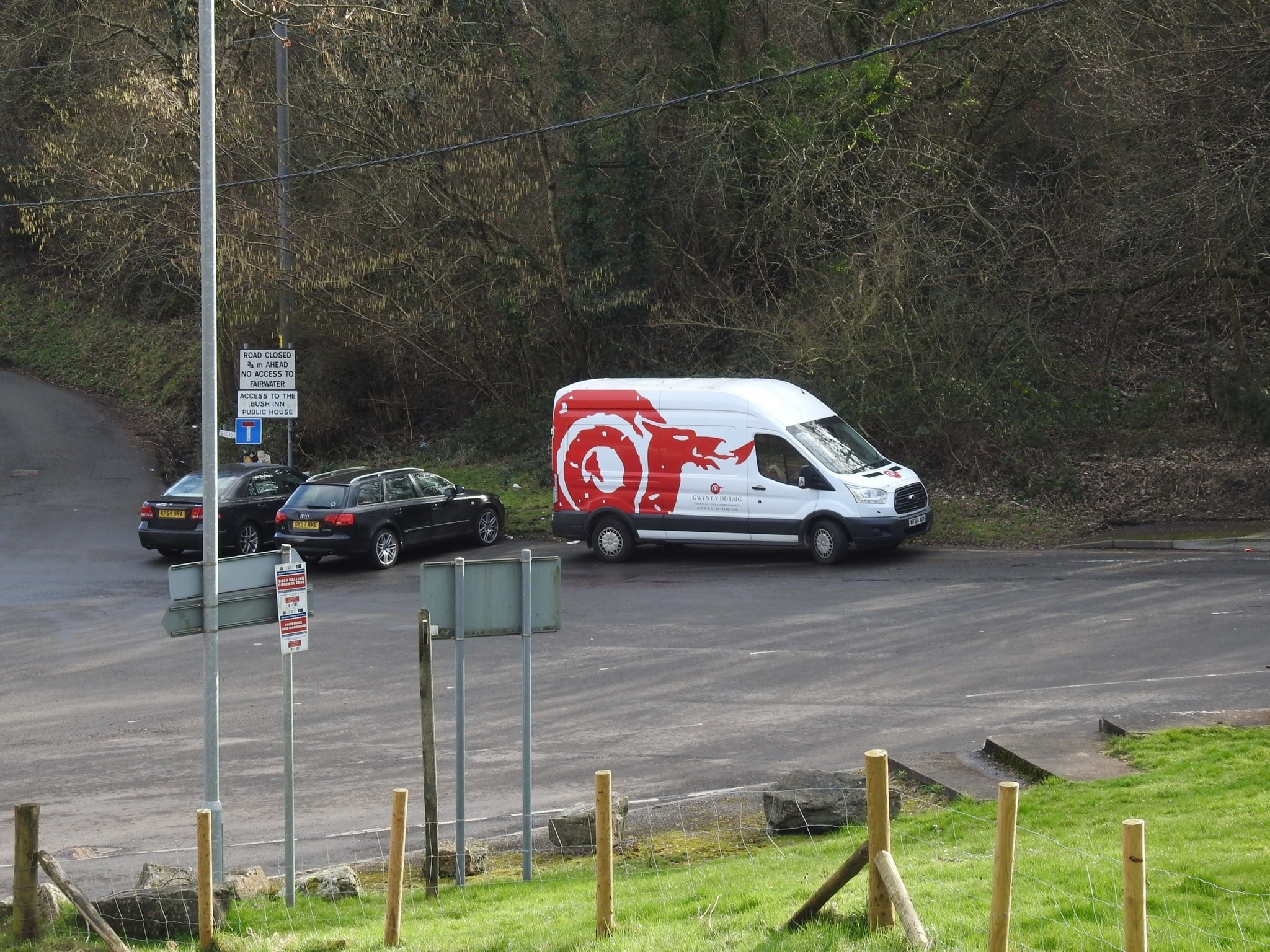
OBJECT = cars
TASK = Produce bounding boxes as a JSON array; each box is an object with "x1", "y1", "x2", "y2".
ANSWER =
[{"x1": 138, "y1": 462, "x2": 312, "y2": 559}]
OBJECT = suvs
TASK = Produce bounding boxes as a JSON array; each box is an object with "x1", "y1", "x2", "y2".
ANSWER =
[{"x1": 271, "y1": 465, "x2": 507, "y2": 565}]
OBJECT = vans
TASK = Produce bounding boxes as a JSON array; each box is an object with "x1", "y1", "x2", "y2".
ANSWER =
[{"x1": 548, "y1": 378, "x2": 937, "y2": 562}]
